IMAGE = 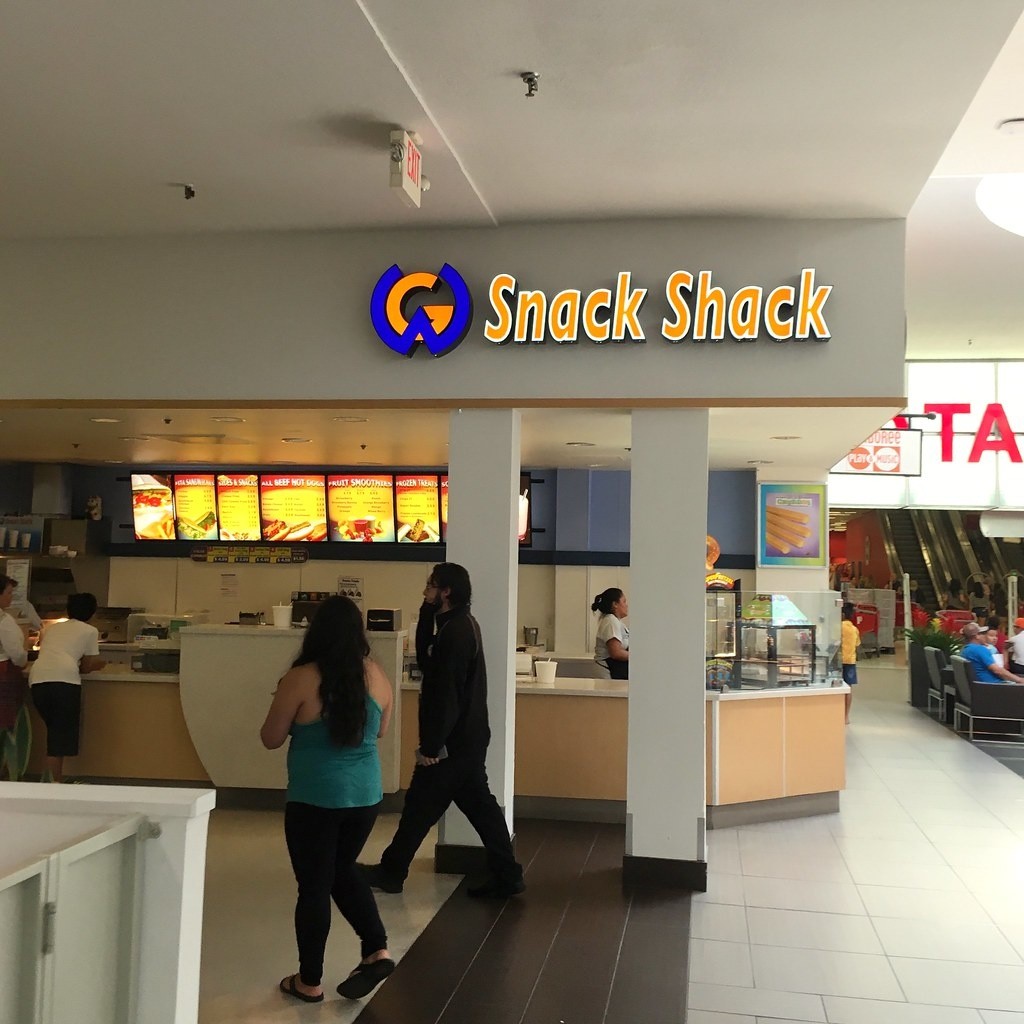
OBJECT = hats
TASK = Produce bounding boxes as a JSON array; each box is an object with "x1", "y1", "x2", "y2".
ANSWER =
[
  {"x1": 963, "y1": 622, "x2": 989, "y2": 636},
  {"x1": 1012, "y1": 617, "x2": 1024, "y2": 629},
  {"x1": 910, "y1": 580, "x2": 918, "y2": 592}
]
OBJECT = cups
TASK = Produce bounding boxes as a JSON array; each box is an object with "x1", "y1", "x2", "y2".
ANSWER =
[
  {"x1": 67, "y1": 550, "x2": 77, "y2": 557},
  {"x1": 355, "y1": 519, "x2": 368, "y2": 531},
  {"x1": 0, "y1": 527, "x2": 7, "y2": 547},
  {"x1": 22, "y1": 534, "x2": 31, "y2": 548},
  {"x1": 524, "y1": 625, "x2": 538, "y2": 646},
  {"x1": 366, "y1": 516, "x2": 375, "y2": 528},
  {"x1": 535, "y1": 661, "x2": 558, "y2": 684},
  {"x1": 9, "y1": 529, "x2": 18, "y2": 547},
  {"x1": 272, "y1": 606, "x2": 293, "y2": 630}
]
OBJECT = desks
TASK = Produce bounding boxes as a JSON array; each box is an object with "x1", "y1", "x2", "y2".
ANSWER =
[{"x1": 942, "y1": 684, "x2": 957, "y2": 723}]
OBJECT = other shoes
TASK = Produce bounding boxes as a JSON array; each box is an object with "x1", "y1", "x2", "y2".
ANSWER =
[
  {"x1": 354, "y1": 861, "x2": 405, "y2": 893},
  {"x1": 490, "y1": 880, "x2": 525, "y2": 895},
  {"x1": 64, "y1": 778, "x2": 86, "y2": 784}
]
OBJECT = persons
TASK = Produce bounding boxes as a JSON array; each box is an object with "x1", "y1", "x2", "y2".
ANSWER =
[
  {"x1": 910, "y1": 581, "x2": 926, "y2": 603},
  {"x1": 591, "y1": 588, "x2": 629, "y2": 681},
  {"x1": 946, "y1": 578, "x2": 1024, "y2": 685},
  {"x1": 0, "y1": 577, "x2": 35, "y2": 736},
  {"x1": 360, "y1": 562, "x2": 526, "y2": 899},
  {"x1": 260, "y1": 595, "x2": 395, "y2": 1003},
  {"x1": 841, "y1": 602, "x2": 862, "y2": 725},
  {"x1": 28, "y1": 593, "x2": 108, "y2": 783},
  {"x1": 885, "y1": 573, "x2": 902, "y2": 594}
]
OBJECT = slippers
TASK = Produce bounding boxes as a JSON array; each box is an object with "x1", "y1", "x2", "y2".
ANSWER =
[
  {"x1": 279, "y1": 973, "x2": 324, "y2": 1002},
  {"x1": 336, "y1": 958, "x2": 396, "y2": 1000}
]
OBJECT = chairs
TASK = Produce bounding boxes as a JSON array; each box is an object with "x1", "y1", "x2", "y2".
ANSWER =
[
  {"x1": 923, "y1": 646, "x2": 954, "y2": 721},
  {"x1": 949, "y1": 655, "x2": 1024, "y2": 742}
]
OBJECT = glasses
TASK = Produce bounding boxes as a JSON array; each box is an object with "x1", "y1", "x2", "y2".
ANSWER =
[
  {"x1": 979, "y1": 632, "x2": 987, "y2": 634},
  {"x1": 426, "y1": 585, "x2": 439, "y2": 592}
]
